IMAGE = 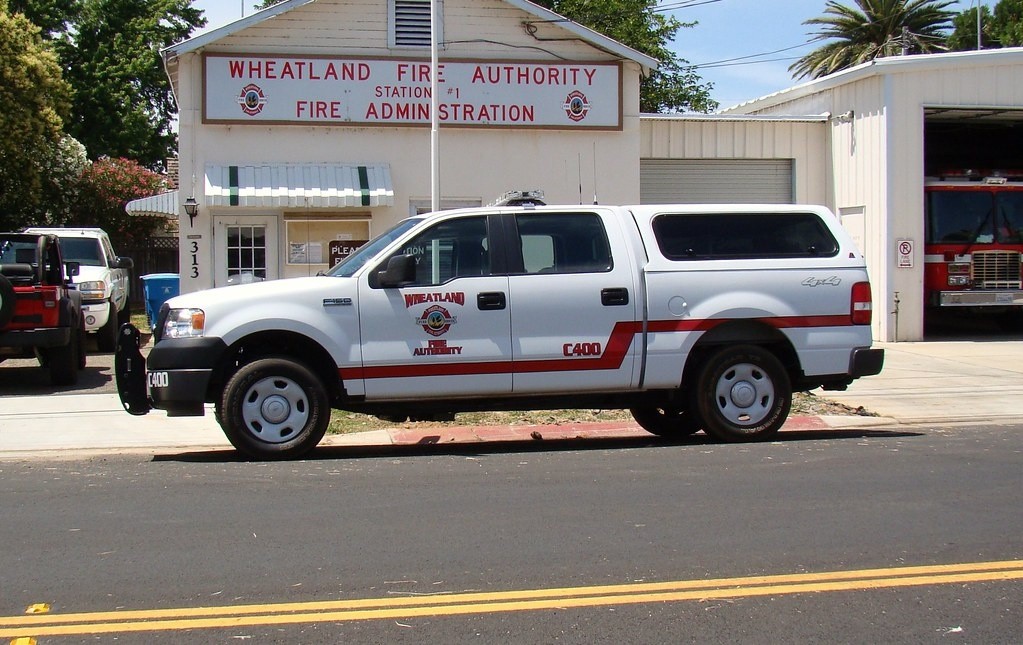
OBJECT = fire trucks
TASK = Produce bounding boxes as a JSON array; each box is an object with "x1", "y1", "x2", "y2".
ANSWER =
[{"x1": 924, "y1": 169, "x2": 1023, "y2": 332}]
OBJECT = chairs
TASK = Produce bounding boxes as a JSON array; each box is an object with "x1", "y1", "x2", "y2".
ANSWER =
[
  {"x1": 564, "y1": 236, "x2": 590, "y2": 268},
  {"x1": 461, "y1": 244, "x2": 490, "y2": 282}
]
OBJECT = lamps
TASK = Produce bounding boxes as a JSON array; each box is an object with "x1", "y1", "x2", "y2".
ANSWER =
[{"x1": 183, "y1": 196, "x2": 199, "y2": 227}]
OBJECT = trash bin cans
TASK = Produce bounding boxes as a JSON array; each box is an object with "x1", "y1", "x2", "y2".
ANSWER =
[{"x1": 139, "y1": 273, "x2": 179, "y2": 328}]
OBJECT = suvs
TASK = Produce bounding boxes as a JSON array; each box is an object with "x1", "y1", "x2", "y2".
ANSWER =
[{"x1": 0, "y1": 232, "x2": 87, "y2": 386}]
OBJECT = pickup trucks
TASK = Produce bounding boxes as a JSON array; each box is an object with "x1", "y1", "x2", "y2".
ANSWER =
[
  {"x1": 23, "y1": 227, "x2": 134, "y2": 353},
  {"x1": 116, "y1": 188, "x2": 885, "y2": 462}
]
